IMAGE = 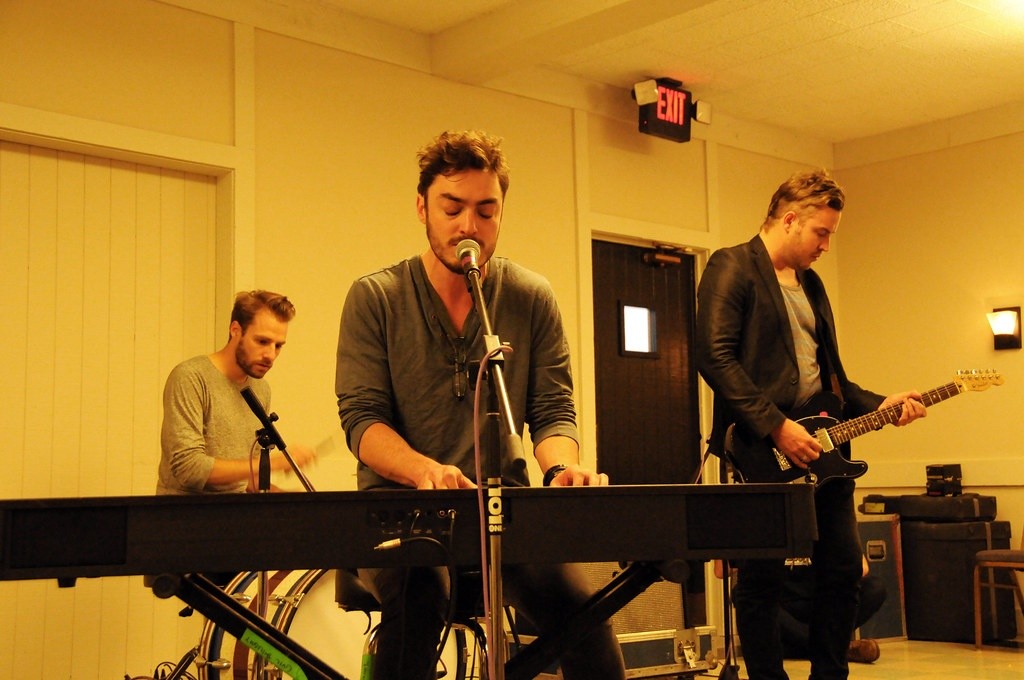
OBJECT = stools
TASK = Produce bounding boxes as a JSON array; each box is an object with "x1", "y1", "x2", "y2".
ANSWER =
[{"x1": 335, "y1": 566, "x2": 490, "y2": 680}]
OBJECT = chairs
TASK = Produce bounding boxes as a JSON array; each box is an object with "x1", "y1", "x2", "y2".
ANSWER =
[{"x1": 973, "y1": 529, "x2": 1024, "y2": 650}]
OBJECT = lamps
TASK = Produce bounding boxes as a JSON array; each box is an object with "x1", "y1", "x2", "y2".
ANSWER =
[
  {"x1": 633, "y1": 79, "x2": 659, "y2": 106},
  {"x1": 692, "y1": 99, "x2": 713, "y2": 125},
  {"x1": 985, "y1": 306, "x2": 1022, "y2": 349}
]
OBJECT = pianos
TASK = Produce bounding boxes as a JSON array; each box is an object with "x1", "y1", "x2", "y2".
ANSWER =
[{"x1": 1, "y1": 480, "x2": 823, "y2": 583}]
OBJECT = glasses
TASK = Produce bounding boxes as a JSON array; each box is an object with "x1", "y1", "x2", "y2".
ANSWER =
[{"x1": 451, "y1": 336, "x2": 467, "y2": 398}]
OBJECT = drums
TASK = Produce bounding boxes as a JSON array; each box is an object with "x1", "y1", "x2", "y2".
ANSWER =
[{"x1": 194, "y1": 560, "x2": 488, "y2": 680}]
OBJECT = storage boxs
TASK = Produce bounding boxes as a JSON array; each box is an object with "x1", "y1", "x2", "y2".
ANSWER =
[
  {"x1": 927, "y1": 481, "x2": 962, "y2": 497},
  {"x1": 883, "y1": 492, "x2": 997, "y2": 522},
  {"x1": 851, "y1": 512, "x2": 910, "y2": 645},
  {"x1": 926, "y1": 463, "x2": 962, "y2": 481},
  {"x1": 505, "y1": 623, "x2": 718, "y2": 680},
  {"x1": 897, "y1": 520, "x2": 1017, "y2": 643}
]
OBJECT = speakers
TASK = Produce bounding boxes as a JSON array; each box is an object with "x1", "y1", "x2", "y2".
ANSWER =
[{"x1": 569, "y1": 563, "x2": 692, "y2": 639}]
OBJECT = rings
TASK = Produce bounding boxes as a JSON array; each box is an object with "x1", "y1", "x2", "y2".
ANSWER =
[{"x1": 802, "y1": 454, "x2": 806, "y2": 460}]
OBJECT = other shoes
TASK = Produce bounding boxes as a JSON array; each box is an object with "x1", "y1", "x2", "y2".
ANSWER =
[{"x1": 848, "y1": 638, "x2": 880, "y2": 663}]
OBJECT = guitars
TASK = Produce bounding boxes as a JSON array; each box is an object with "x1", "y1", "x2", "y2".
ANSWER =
[{"x1": 725, "y1": 367, "x2": 1007, "y2": 496}]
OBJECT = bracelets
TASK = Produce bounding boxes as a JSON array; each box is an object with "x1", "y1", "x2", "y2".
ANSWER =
[{"x1": 543, "y1": 464, "x2": 566, "y2": 486}]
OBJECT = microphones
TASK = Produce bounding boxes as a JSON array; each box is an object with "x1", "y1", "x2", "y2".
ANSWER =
[{"x1": 456, "y1": 238, "x2": 482, "y2": 282}]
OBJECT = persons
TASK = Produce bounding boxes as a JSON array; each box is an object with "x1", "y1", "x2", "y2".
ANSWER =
[
  {"x1": 336, "y1": 130, "x2": 626, "y2": 679},
  {"x1": 143, "y1": 292, "x2": 316, "y2": 588},
  {"x1": 694, "y1": 168, "x2": 926, "y2": 680}
]
